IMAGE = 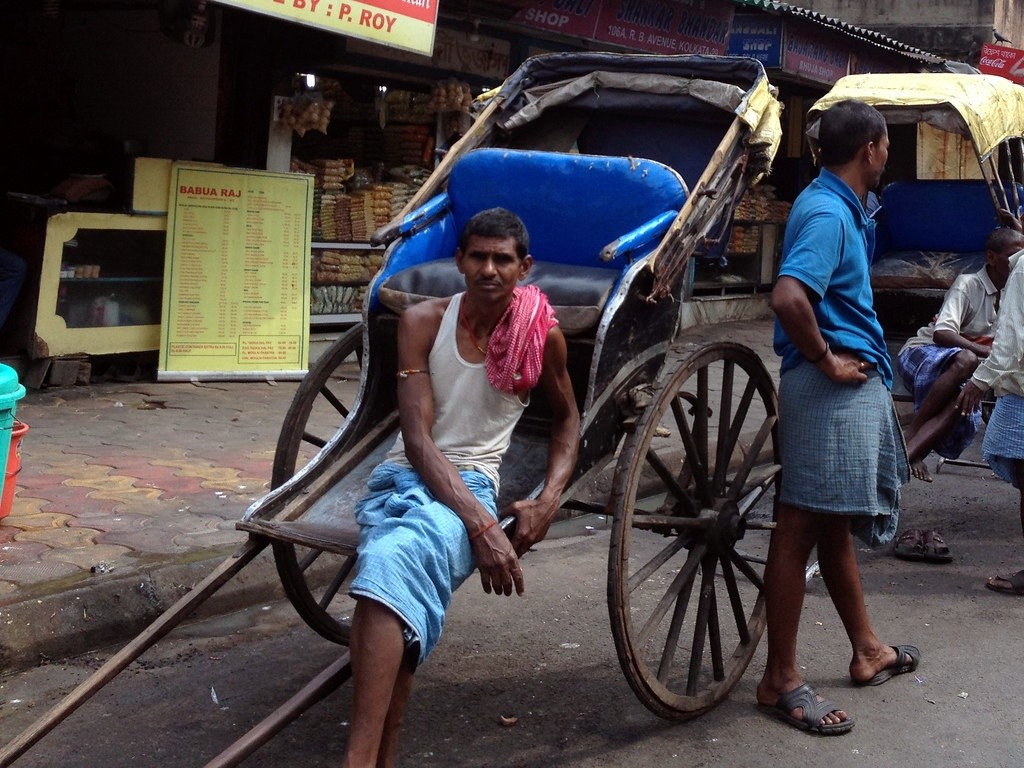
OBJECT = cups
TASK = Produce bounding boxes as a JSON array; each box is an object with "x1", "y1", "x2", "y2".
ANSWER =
[{"x1": 102, "y1": 301, "x2": 120, "y2": 326}]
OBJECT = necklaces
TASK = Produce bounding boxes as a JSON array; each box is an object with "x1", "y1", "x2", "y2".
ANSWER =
[{"x1": 461, "y1": 293, "x2": 487, "y2": 355}]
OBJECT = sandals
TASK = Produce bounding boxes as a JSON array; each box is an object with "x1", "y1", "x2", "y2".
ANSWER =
[
  {"x1": 986, "y1": 570, "x2": 1024, "y2": 597},
  {"x1": 922, "y1": 530, "x2": 953, "y2": 564},
  {"x1": 893, "y1": 529, "x2": 924, "y2": 560}
]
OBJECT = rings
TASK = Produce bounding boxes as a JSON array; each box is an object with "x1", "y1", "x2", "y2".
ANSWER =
[{"x1": 860, "y1": 362, "x2": 865, "y2": 369}]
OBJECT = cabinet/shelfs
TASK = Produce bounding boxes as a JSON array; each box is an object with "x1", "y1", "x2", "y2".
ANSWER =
[
  {"x1": 692, "y1": 219, "x2": 788, "y2": 295},
  {"x1": 310, "y1": 243, "x2": 387, "y2": 323}
]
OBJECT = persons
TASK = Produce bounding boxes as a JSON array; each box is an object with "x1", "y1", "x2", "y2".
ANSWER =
[
  {"x1": 342, "y1": 208, "x2": 580, "y2": 768},
  {"x1": 895, "y1": 227, "x2": 1024, "y2": 482},
  {"x1": 0, "y1": 163, "x2": 111, "y2": 332},
  {"x1": 955, "y1": 212, "x2": 1024, "y2": 595},
  {"x1": 753, "y1": 96, "x2": 922, "y2": 735}
]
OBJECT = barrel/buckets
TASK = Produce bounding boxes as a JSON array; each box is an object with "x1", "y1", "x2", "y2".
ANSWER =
[{"x1": 0, "y1": 421, "x2": 29, "y2": 519}]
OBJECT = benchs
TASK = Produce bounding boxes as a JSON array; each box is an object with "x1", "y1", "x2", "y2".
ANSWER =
[
  {"x1": 376, "y1": 148, "x2": 690, "y2": 333},
  {"x1": 869, "y1": 179, "x2": 1024, "y2": 290}
]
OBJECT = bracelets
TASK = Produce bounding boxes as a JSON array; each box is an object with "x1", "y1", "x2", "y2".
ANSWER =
[
  {"x1": 470, "y1": 520, "x2": 496, "y2": 540},
  {"x1": 806, "y1": 340, "x2": 829, "y2": 363}
]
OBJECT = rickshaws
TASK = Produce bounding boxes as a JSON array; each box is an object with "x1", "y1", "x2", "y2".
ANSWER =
[
  {"x1": 805, "y1": 73, "x2": 1024, "y2": 493},
  {"x1": 1, "y1": 47, "x2": 780, "y2": 768}
]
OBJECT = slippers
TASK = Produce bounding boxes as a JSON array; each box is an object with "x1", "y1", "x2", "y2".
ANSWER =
[
  {"x1": 858, "y1": 644, "x2": 921, "y2": 686},
  {"x1": 755, "y1": 681, "x2": 856, "y2": 734}
]
